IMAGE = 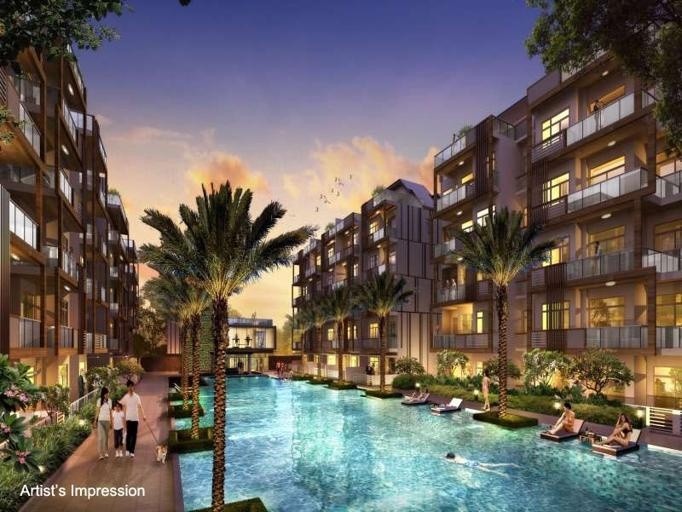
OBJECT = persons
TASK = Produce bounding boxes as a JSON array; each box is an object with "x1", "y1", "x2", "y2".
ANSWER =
[
  {"x1": 288, "y1": 368, "x2": 293, "y2": 381},
  {"x1": 480, "y1": 370, "x2": 492, "y2": 412},
  {"x1": 280, "y1": 361, "x2": 284, "y2": 375},
  {"x1": 449, "y1": 278, "x2": 457, "y2": 300},
  {"x1": 589, "y1": 99, "x2": 599, "y2": 132},
  {"x1": 409, "y1": 387, "x2": 429, "y2": 403},
  {"x1": 405, "y1": 386, "x2": 423, "y2": 404},
  {"x1": 590, "y1": 241, "x2": 604, "y2": 276},
  {"x1": 440, "y1": 451, "x2": 521, "y2": 479},
  {"x1": 545, "y1": 402, "x2": 576, "y2": 436},
  {"x1": 596, "y1": 101, "x2": 607, "y2": 129},
  {"x1": 113, "y1": 379, "x2": 146, "y2": 459},
  {"x1": 370, "y1": 363, "x2": 375, "y2": 375},
  {"x1": 91, "y1": 386, "x2": 114, "y2": 460},
  {"x1": 108, "y1": 401, "x2": 126, "y2": 459},
  {"x1": 275, "y1": 360, "x2": 281, "y2": 377},
  {"x1": 595, "y1": 412, "x2": 633, "y2": 448},
  {"x1": 444, "y1": 279, "x2": 450, "y2": 302},
  {"x1": 365, "y1": 361, "x2": 373, "y2": 375}
]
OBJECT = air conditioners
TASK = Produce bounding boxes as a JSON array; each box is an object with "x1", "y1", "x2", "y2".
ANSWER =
[{"x1": 153, "y1": 443, "x2": 168, "y2": 464}]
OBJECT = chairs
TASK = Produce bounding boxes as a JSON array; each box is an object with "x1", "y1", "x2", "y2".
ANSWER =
[{"x1": 99, "y1": 451, "x2": 134, "y2": 460}]
